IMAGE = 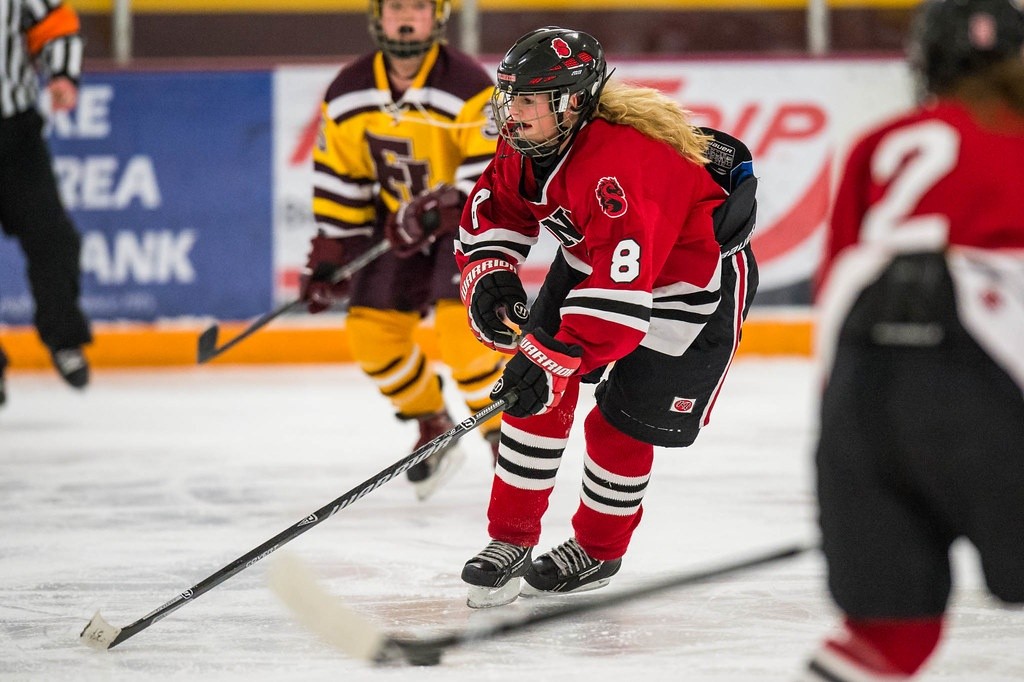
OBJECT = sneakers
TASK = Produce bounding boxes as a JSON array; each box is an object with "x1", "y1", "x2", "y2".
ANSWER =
[
  {"x1": 485, "y1": 430, "x2": 501, "y2": 469},
  {"x1": 518, "y1": 537, "x2": 622, "y2": 598},
  {"x1": 461, "y1": 537, "x2": 533, "y2": 609},
  {"x1": 406, "y1": 411, "x2": 460, "y2": 498}
]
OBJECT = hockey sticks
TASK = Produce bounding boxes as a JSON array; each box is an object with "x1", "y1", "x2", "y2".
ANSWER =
[
  {"x1": 370, "y1": 538, "x2": 818, "y2": 667},
  {"x1": 78, "y1": 391, "x2": 521, "y2": 648},
  {"x1": 195, "y1": 237, "x2": 390, "y2": 363}
]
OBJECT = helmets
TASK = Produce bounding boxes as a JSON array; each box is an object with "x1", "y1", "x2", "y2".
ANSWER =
[
  {"x1": 491, "y1": 26, "x2": 616, "y2": 158},
  {"x1": 367, "y1": 0, "x2": 451, "y2": 27},
  {"x1": 911, "y1": 0, "x2": 1024, "y2": 108}
]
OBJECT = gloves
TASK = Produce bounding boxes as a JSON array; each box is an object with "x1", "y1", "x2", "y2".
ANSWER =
[
  {"x1": 460, "y1": 258, "x2": 530, "y2": 355},
  {"x1": 489, "y1": 327, "x2": 583, "y2": 419},
  {"x1": 303, "y1": 261, "x2": 352, "y2": 314},
  {"x1": 387, "y1": 183, "x2": 461, "y2": 260}
]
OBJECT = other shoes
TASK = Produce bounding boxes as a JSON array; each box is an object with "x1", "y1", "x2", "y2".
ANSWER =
[{"x1": 49, "y1": 347, "x2": 88, "y2": 388}]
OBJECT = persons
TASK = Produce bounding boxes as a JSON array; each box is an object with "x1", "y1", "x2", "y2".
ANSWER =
[
  {"x1": 0, "y1": 0, "x2": 95, "y2": 404},
  {"x1": 304, "y1": 0, "x2": 760, "y2": 609},
  {"x1": 799, "y1": 0, "x2": 1023, "y2": 682}
]
